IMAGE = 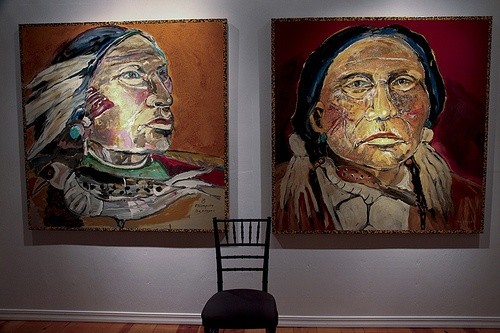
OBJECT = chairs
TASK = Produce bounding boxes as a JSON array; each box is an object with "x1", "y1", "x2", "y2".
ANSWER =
[{"x1": 200, "y1": 216, "x2": 279, "y2": 333}]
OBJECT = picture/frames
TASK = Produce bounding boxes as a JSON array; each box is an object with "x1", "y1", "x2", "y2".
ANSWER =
[
  {"x1": 18, "y1": 18, "x2": 230, "y2": 234},
  {"x1": 271, "y1": 16, "x2": 492, "y2": 235}
]
